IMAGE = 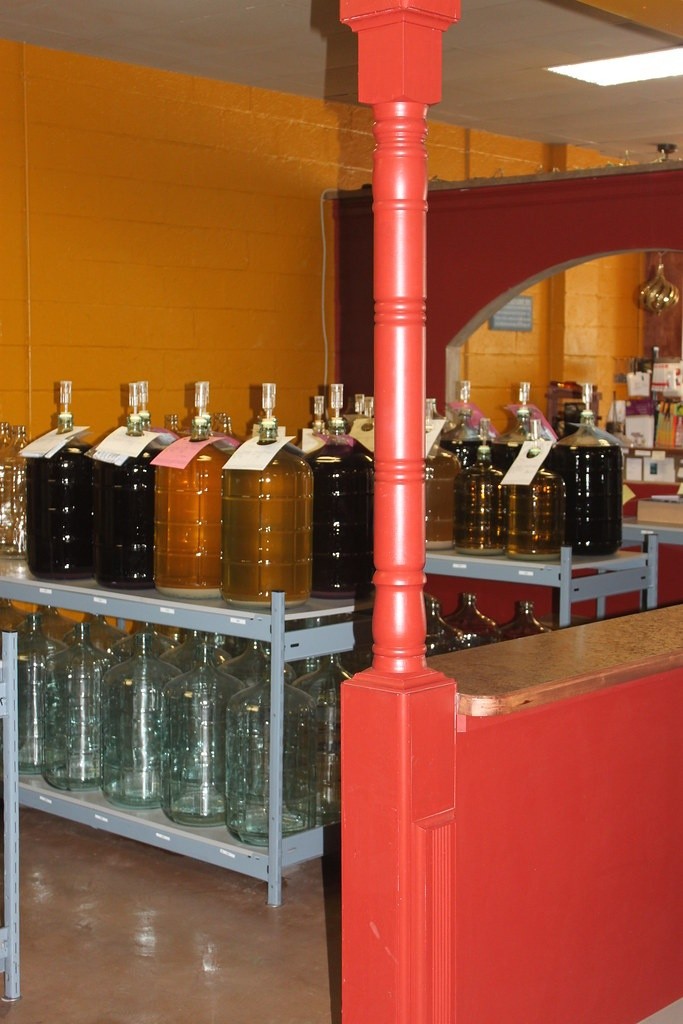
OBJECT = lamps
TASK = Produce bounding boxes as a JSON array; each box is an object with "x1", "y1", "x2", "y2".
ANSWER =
[{"x1": 636, "y1": 250, "x2": 680, "y2": 321}]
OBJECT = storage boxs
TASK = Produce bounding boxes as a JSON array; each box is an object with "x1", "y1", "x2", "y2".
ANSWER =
[{"x1": 651, "y1": 363, "x2": 683, "y2": 392}]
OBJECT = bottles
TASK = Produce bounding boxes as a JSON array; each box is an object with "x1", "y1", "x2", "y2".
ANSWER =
[{"x1": 0, "y1": 376, "x2": 625, "y2": 848}]
